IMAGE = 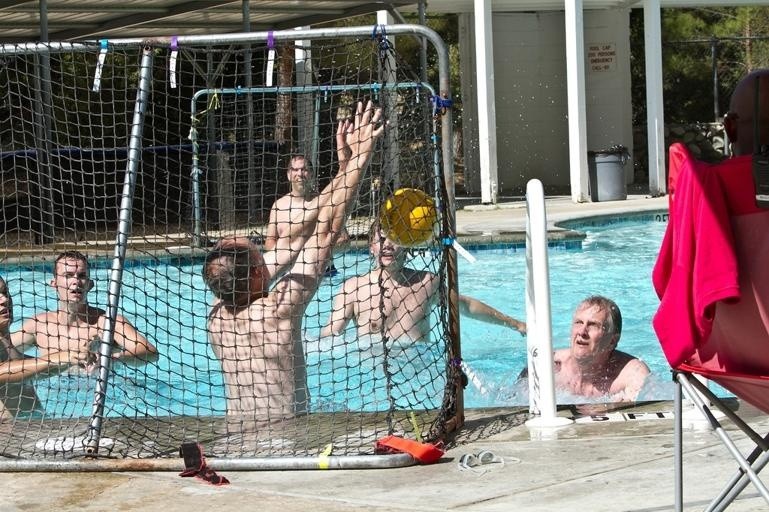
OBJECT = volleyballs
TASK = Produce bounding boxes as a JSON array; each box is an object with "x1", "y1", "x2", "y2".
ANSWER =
[{"x1": 378, "y1": 187, "x2": 436, "y2": 247}]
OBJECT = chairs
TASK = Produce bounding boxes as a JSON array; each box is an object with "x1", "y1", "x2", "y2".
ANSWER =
[{"x1": 649, "y1": 140, "x2": 769, "y2": 511}]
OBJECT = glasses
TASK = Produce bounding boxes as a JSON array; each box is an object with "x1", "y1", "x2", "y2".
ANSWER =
[{"x1": 460, "y1": 450, "x2": 505, "y2": 477}]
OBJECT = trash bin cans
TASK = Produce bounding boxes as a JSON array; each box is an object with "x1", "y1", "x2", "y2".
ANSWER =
[{"x1": 587, "y1": 150, "x2": 627, "y2": 202}]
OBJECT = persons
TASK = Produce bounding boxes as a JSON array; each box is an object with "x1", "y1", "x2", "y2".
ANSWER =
[
  {"x1": 516, "y1": 295, "x2": 653, "y2": 402},
  {"x1": 200, "y1": 100, "x2": 383, "y2": 418},
  {"x1": 262, "y1": 153, "x2": 353, "y2": 281},
  {"x1": 321, "y1": 212, "x2": 526, "y2": 348},
  {"x1": 0, "y1": 276, "x2": 98, "y2": 425},
  {"x1": 5, "y1": 251, "x2": 158, "y2": 380},
  {"x1": 720, "y1": 67, "x2": 767, "y2": 211}
]
